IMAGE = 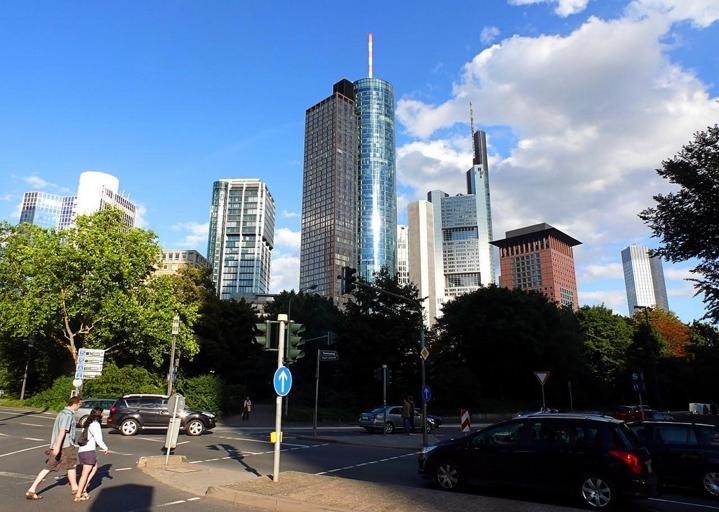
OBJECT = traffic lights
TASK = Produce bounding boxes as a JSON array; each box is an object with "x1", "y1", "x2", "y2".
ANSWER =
[
  {"x1": 287, "y1": 322, "x2": 307, "y2": 363},
  {"x1": 345, "y1": 267, "x2": 356, "y2": 292},
  {"x1": 251, "y1": 320, "x2": 279, "y2": 352}
]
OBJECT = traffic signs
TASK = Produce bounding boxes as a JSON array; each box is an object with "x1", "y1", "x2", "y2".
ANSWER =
[
  {"x1": 75, "y1": 348, "x2": 106, "y2": 380},
  {"x1": 321, "y1": 350, "x2": 338, "y2": 362}
]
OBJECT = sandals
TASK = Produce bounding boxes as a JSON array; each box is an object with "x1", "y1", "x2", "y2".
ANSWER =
[
  {"x1": 25, "y1": 490, "x2": 44, "y2": 500},
  {"x1": 72, "y1": 488, "x2": 90, "y2": 503}
]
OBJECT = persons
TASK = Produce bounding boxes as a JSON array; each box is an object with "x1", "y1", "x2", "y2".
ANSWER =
[
  {"x1": 25, "y1": 397, "x2": 111, "y2": 502},
  {"x1": 242, "y1": 397, "x2": 252, "y2": 421},
  {"x1": 401, "y1": 396, "x2": 415, "y2": 436}
]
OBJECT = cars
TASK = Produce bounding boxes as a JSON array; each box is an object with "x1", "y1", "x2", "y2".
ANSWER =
[
  {"x1": 414, "y1": 414, "x2": 656, "y2": 512},
  {"x1": 625, "y1": 420, "x2": 719, "y2": 501},
  {"x1": 614, "y1": 404, "x2": 675, "y2": 421},
  {"x1": 359, "y1": 406, "x2": 441, "y2": 434},
  {"x1": 74, "y1": 393, "x2": 216, "y2": 436}
]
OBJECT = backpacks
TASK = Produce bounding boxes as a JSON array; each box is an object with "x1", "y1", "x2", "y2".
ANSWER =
[{"x1": 78, "y1": 419, "x2": 94, "y2": 446}]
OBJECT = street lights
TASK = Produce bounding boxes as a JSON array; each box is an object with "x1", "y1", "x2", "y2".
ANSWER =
[
  {"x1": 382, "y1": 365, "x2": 387, "y2": 435},
  {"x1": 166, "y1": 315, "x2": 180, "y2": 395}
]
